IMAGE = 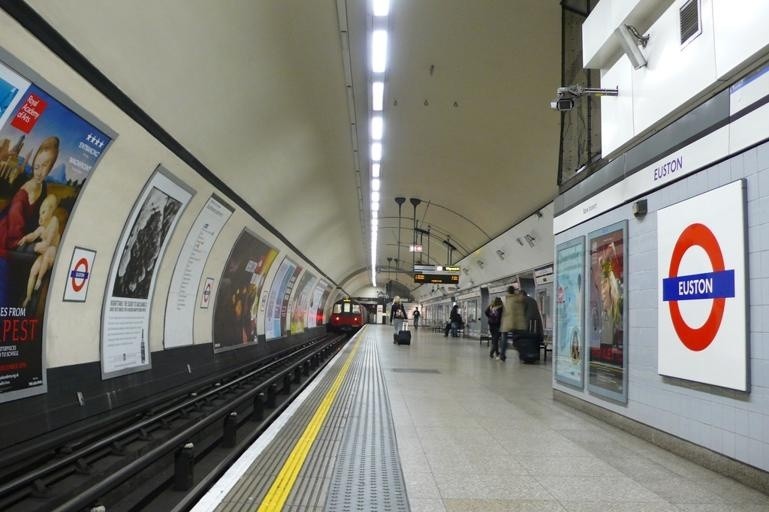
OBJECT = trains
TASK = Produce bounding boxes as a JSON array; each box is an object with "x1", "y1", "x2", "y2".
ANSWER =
[{"x1": 330, "y1": 299, "x2": 369, "y2": 332}]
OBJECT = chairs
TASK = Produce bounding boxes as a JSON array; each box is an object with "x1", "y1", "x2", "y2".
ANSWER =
[
  {"x1": 539, "y1": 327, "x2": 554, "y2": 363},
  {"x1": 429, "y1": 320, "x2": 467, "y2": 338},
  {"x1": 479, "y1": 329, "x2": 493, "y2": 347}
]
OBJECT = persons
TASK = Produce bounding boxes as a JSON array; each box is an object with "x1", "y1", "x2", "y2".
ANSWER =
[
  {"x1": 517, "y1": 290, "x2": 544, "y2": 342},
  {"x1": 390, "y1": 296, "x2": 408, "y2": 345},
  {"x1": 485, "y1": 297, "x2": 504, "y2": 358},
  {"x1": 598, "y1": 257, "x2": 623, "y2": 332},
  {"x1": 444, "y1": 304, "x2": 463, "y2": 337},
  {"x1": 413, "y1": 307, "x2": 420, "y2": 330},
  {"x1": 496, "y1": 286, "x2": 527, "y2": 361},
  {"x1": 571, "y1": 331, "x2": 580, "y2": 359}
]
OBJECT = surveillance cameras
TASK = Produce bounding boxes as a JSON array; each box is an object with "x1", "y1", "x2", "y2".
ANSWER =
[
  {"x1": 551, "y1": 99, "x2": 575, "y2": 111},
  {"x1": 614, "y1": 24, "x2": 647, "y2": 69}
]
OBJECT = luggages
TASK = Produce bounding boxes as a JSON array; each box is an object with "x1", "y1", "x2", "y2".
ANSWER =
[
  {"x1": 398, "y1": 320, "x2": 411, "y2": 345},
  {"x1": 518, "y1": 320, "x2": 541, "y2": 363}
]
